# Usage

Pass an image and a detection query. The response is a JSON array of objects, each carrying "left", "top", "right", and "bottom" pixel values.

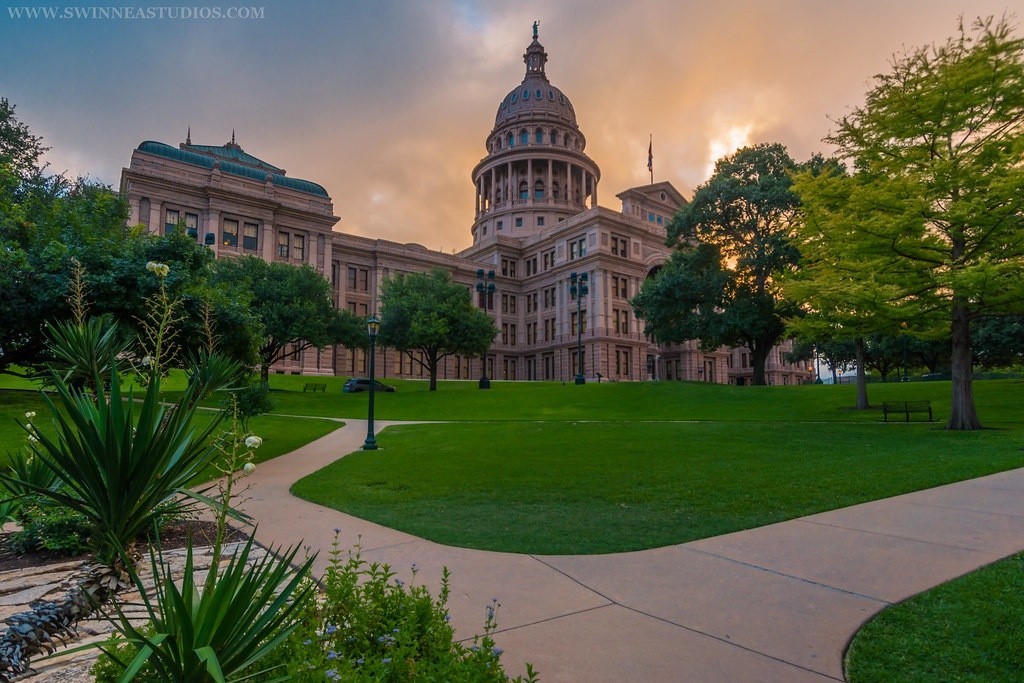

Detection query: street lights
[
  {"left": 363, "top": 316, "right": 381, "bottom": 450},
  {"left": 476, "top": 269, "right": 496, "bottom": 389},
  {"left": 570, "top": 272, "right": 588, "bottom": 384}
]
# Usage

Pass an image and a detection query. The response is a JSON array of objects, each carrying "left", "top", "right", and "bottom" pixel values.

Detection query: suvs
[{"left": 343, "top": 378, "right": 396, "bottom": 392}]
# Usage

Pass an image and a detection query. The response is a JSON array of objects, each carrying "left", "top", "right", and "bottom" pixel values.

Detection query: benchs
[
  {"left": 882, "top": 401, "right": 934, "bottom": 422},
  {"left": 303, "top": 383, "right": 326, "bottom": 393}
]
